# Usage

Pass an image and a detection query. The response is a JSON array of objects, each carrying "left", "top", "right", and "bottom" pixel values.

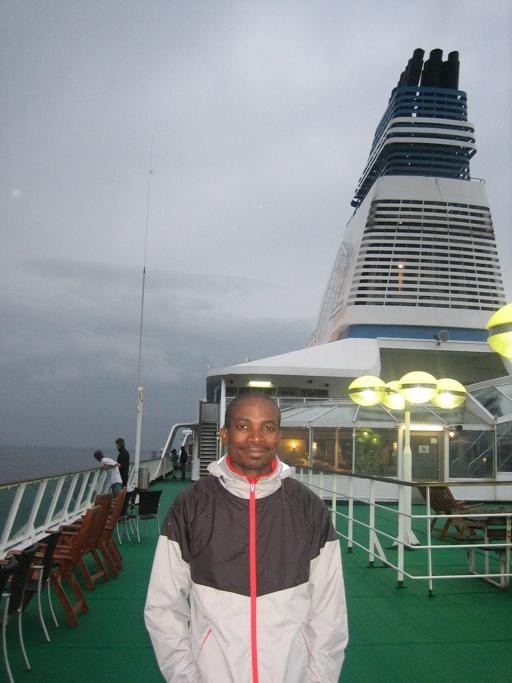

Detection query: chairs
[
  {"left": 417, "top": 480, "right": 512, "bottom": 542},
  {"left": 0, "top": 485, "right": 163, "bottom": 683}
]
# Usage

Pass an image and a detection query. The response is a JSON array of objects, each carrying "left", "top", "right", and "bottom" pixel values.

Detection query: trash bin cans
[{"left": 138, "top": 468, "right": 149, "bottom": 489}]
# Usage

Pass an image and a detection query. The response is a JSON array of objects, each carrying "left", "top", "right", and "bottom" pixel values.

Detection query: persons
[
  {"left": 94, "top": 450, "right": 124, "bottom": 497},
  {"left": 115, "top": 437, "right": 130, "bottom": 504},
  {"left": 142, "top": 390, "right": 349, "bottom": 683},
  {"left": 171, "top": 448, "right": 179, "bottom": 479},
  {"left": 179, "top": 445, "right": 188, "bottom": 479}
]
